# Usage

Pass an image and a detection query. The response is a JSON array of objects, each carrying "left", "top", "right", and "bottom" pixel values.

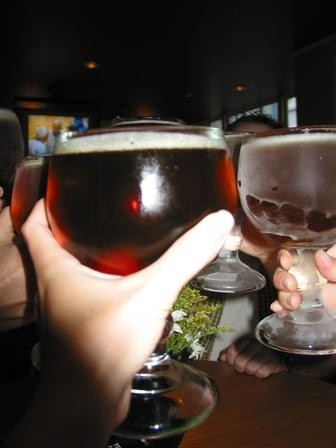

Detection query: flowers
[{"left": 158, "top": 284, "right": 239, "bottom": 361}]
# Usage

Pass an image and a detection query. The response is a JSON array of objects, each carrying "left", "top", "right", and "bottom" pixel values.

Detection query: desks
[{"left": 0, "top": 359, "right": 336, "bottom": 448}]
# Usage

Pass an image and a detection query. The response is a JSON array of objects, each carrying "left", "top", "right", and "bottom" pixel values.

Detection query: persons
[
  {"left": 46, "top": 120, "right": 66, "bottom": 155},
  {"left": 29, "top": 126, "right": 49, "bottom": 155},
  {"left": 21, "top": 197, "right": 235, "bottom": 448},
  {"left": 196, "top": 110, "right": 288, "bottom": 379}
]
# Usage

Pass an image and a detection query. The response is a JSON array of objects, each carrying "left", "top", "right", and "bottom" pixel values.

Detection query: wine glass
[
  {"left": 236, "top": 125, "right": 336, "bottom": 355},
  {"left": 41, "top": 125, "right": 237, "bottom": 441},
  {"left": 189, "top": 131, "right": 267, "bottom": 294},
  {"left": 9, "top": 154, "right": 49, "bottom": 371}
]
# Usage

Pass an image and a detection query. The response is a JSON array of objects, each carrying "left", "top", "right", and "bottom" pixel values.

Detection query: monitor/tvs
[{"left": 17, "top": 107, "right": 98, "bottom": 157}]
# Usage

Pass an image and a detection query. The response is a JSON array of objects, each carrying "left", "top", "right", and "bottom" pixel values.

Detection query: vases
[{"left": 106, "top": 376, "right": 186, "bottom": 448}]
